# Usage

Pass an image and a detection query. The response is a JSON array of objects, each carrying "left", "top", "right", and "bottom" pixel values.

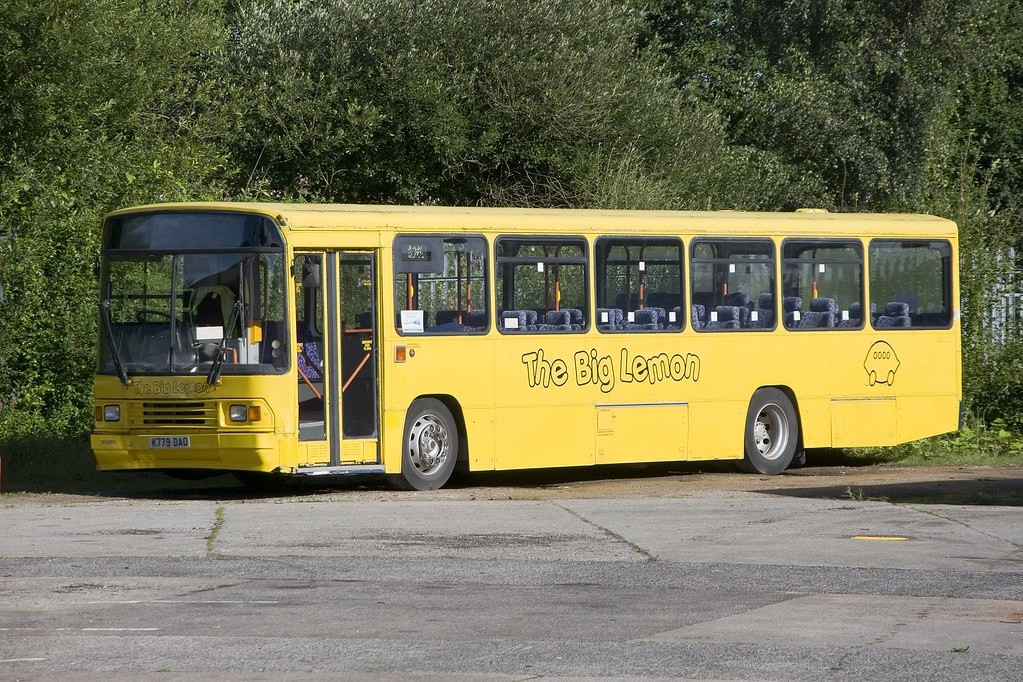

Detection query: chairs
[
  {"left": 260, "top": 319, "right": 313, "bottom": 348},
  {"left": 351, "top": 291, "right": 914, "bottom": 330},
  {"left": 189, "top": 284, "right": 237, "bottom": 339}
]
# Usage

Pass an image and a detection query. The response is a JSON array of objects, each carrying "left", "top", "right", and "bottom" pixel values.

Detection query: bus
[{"left": 90, "top": 200, "right": 964, "bottom": 490}]
